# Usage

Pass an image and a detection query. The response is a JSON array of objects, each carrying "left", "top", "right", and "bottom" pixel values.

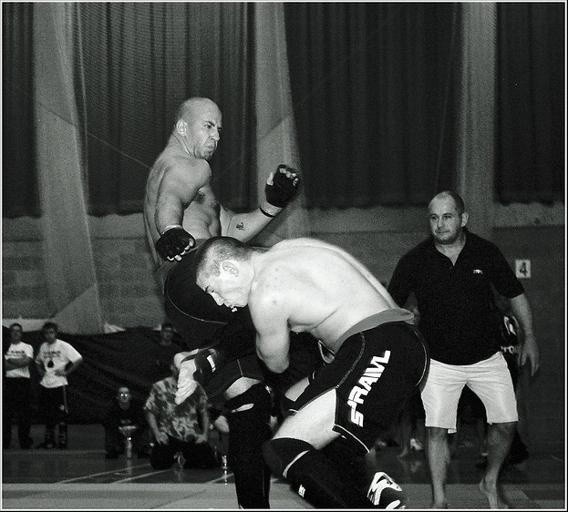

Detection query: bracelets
[{"left": 258, "top": 206, "right": 276, "bottom": 218}]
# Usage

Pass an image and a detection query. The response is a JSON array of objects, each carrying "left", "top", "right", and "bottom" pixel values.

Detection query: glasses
[{"left": 117, "top": 391, "right": 129, "bottom": 398}]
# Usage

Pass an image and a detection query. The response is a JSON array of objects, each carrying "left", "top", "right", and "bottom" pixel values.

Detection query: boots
[
  {"left": 58, "top": 431, "right": 66, "bottom": 449},
  {"left": 44, "top": 428, "right": 55, "bottom": 449}
]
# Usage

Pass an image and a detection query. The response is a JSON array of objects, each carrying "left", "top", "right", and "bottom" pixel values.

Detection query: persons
[
  {"left": 3, "top": 324, "right": 34, "bottom": 450},
  {"left": 387, "top": 191, "right": 541, "bottom": 509},
  {"left": 195, "top": 238, "right": 429, "bottom": 509},
  {"left": 365, "top": 312, "right": 532, "bottom": 463},
  {"left": 107, "top": 323, "right": 231, "bottom": 472},
  {"left": 34, "top": 322, "right": 83, "bottom": 449},
  {"left": 143, "top": 96, "right": 300, "bottom": 509}
]
